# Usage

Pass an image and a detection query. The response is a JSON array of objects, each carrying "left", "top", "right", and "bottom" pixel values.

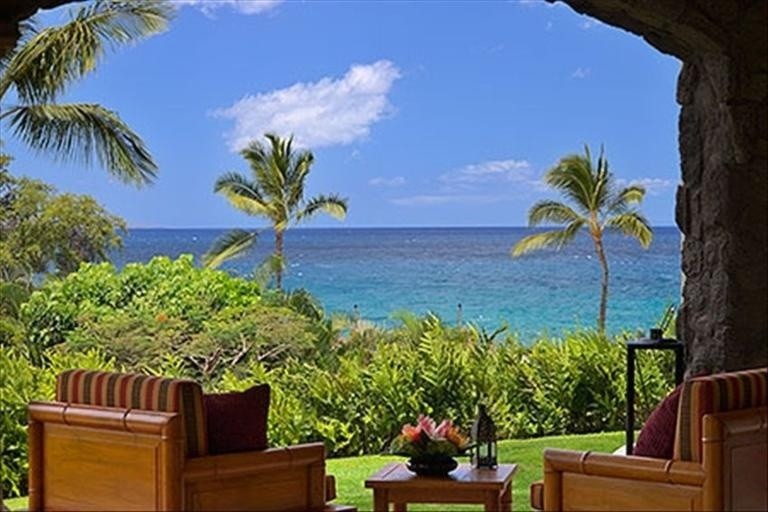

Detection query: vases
[{"left": 405, "top": 455, "right": 459, "bottom": 476}]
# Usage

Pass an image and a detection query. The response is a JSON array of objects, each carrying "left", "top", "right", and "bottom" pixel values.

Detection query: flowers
[{"left": 386, "top": 413, "right": 480, "bottom": 460}]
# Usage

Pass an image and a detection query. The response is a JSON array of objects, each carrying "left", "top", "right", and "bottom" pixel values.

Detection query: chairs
[
  {"left": 529, "top": 367, "right": 768, "bottom": 512},
  {"left": 26, "top": 366, "right": 355, "bottom": 512}
]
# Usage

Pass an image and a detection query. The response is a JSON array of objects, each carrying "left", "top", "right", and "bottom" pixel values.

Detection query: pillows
[
  {"left": 203, "top": 381, "right": 271, "bottom": 457},
  {"left": 631, "top": 383, "right": 682, "bottom": 458}
]
